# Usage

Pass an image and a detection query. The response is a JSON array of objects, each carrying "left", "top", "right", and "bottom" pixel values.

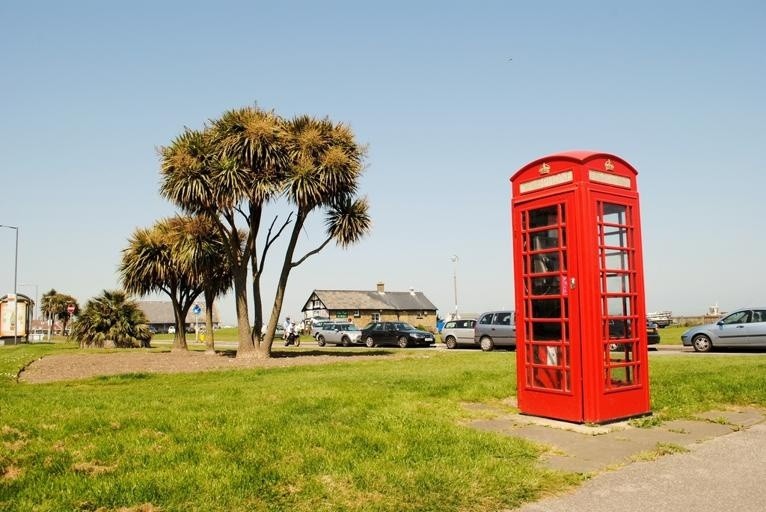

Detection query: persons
[
  {"left": 752, "top": 313, "right": 760, "bottom": 322},
  {"left": 285, "top": 321, "right": 297, "bottom": 341},
  {"left": 283, "top": 317, "right": 291, "bottom": 338}
]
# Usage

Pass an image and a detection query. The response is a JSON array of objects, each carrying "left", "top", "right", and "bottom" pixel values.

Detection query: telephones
[{"left": 533, "top": 235, "right": 561, "bottom": 287}]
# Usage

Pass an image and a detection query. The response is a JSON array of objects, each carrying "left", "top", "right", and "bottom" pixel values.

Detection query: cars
[
  {"left": 311, "top": 319, "right": 435, "bottom": 347},
  {"left": 603, "top": 315, "right": 660, "bottom": 351},
  {"left": 148, "top": 325, "right": 207, "bottom": 334},
  {"left": 260, "top": 323, "right": 287, "bottom": 340},
  {"left": 682, "top": 308, "right": 766, "bottom": 354}
]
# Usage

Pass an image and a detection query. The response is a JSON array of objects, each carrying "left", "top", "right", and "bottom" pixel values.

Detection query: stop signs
[{"left": 66, "top": 303, "right": 75, "bottom": 313}]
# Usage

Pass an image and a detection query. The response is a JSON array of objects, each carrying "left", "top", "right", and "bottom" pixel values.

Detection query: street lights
[
  {"left": 0, "top": 225, "right": 18, "bottom": 345},
  {"left": 450, "top": 254, "right": 459, "bottom": 319}
]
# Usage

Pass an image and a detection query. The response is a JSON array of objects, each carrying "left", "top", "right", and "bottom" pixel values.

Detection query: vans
[
  {"left": 441, "top": 318, "right": 480, "bottom": 349},
  {"left": 474, "top": 311, "right": 516, "bottom": 352}
]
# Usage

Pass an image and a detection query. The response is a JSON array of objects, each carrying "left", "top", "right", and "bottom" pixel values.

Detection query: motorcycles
[{"left": 287, "top": 329, "right": 300, "bottom": 346}]
